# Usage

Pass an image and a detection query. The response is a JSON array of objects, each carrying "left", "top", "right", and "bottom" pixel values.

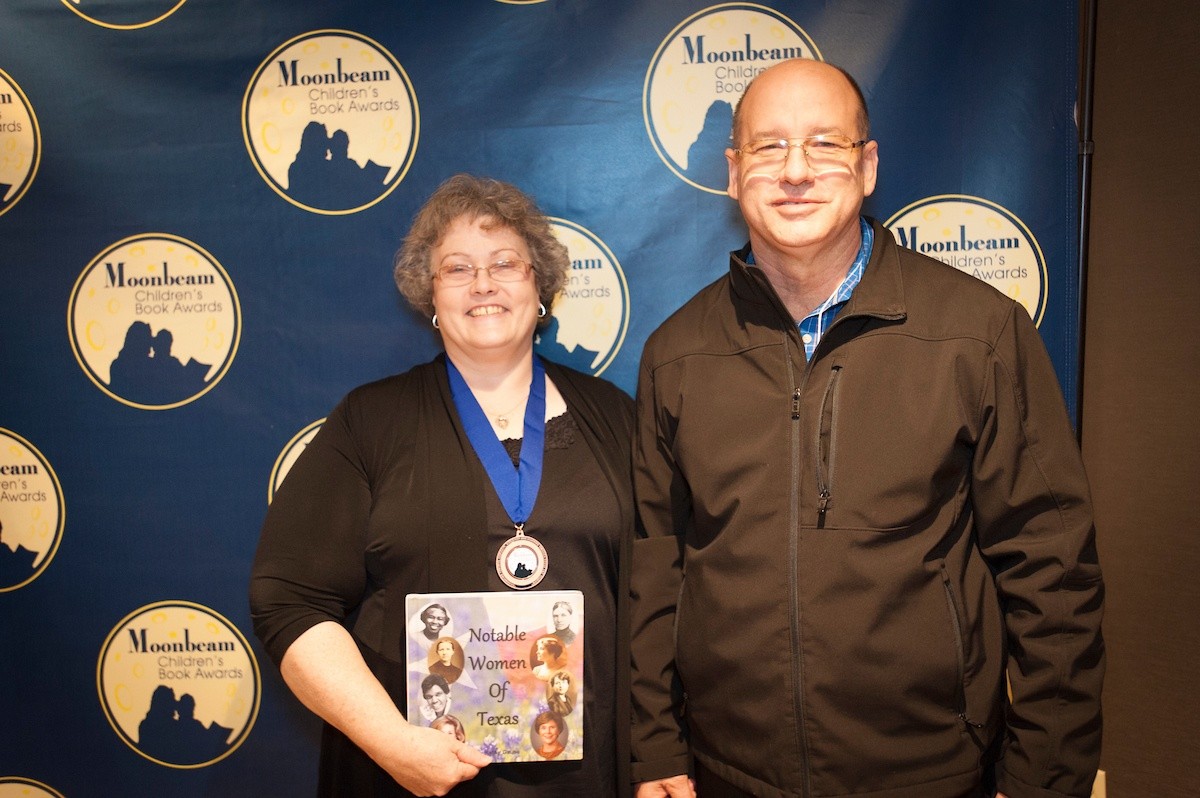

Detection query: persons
[
  {"left": 547, "top": 672, "right": 574, "bottom": 717},
  {"left": 429, "top": 639, "right": 463, "bottom": 684},
  {"left": 432, "top": 714, "right": 465, "bottom": 742},
  {"left": 632, "top": 58, "right": 1106, "bottom": 798},
  {"left": 551, "top": 601, "right": 577, "bottom": 645},
  {"left": 533, "top": 638, "right": 563, "bottom": 681},
  {"left": 251, "top": 174, "right": 634, "bottom": 798},
  {"left": 420, "top": 604, "right": 450, "bottom": 640},
  {"left": 420, "top": 672, "right": 452, "bottom": 722},
  {"left": 535, "top": 711, "right": 565, "bottom": 759}
]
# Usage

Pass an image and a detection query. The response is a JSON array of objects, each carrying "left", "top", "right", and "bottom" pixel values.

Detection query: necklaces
[{"left": 483, "top": 396, "right": 529, "bottom": 429}]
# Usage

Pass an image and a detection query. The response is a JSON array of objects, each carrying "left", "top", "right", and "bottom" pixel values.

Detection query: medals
[{"left": 495, "top": 529, "right": 548, "bottom": 590}]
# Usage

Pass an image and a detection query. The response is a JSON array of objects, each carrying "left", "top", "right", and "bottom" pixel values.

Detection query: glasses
[
  {"left": 733, "top": 136, "right": 867, "bottom": 175},
  {"left": 430, "top": 259, "right": 538, "bottom": 289}
]
